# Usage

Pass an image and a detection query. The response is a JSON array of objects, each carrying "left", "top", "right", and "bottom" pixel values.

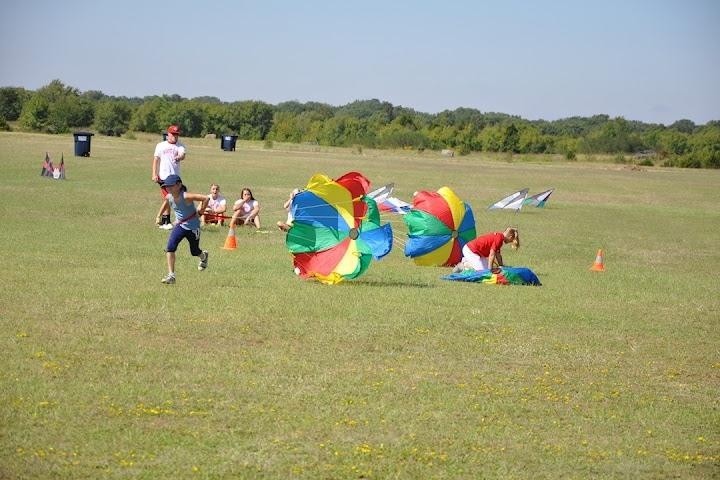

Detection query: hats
[
  {"left": 167, "top": 126, "right": 181, "bottom": 134},
  {"left": 160, "top": 175, "right": 179, "bottom": 188}
]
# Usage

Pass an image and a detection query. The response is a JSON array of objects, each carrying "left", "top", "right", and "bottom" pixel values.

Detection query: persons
[
  {"left": 451, "top": 227, "right": 520, "bottom": 277},
  {"left": 196, "top": 183, "right": 227, "bottom": 228},
  {"left": 276, "top": 187, "right": 301, "bottom": 235},
  {"left": 151, "top": 125, "right": 187, "bottom": 225},
  {"left": 228, "top": 188, "right": 265, "bottom": 230},
  {"left": 155, "top": 174, "right": 212, "bottom": 286}
]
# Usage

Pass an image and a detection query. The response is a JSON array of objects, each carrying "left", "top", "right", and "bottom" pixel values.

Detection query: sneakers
[
  {"left": 161, "top": 275, "right": 175, "bottom": 284},
  {"left": 198, "top": 250, "right": 208, "bottom": 271},
  {"left": 159, "top": 223, "right": 173, "bottom": 230}
]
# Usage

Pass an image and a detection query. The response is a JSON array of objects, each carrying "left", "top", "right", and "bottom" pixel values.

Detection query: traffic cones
[
  {"left": 219, "top": 223, "right": 239, "bottom": 251},
  {"left": 588, "top": 248, "right": 608, "bottom": 272}
]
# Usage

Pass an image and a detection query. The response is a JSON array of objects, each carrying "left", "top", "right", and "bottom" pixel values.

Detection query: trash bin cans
[
  {"left": 74, "top": 132, "right": 94, "bottom": 157},
  {"left": 221, "top": 134, "right": 238, "bottom": 151},
  {"left": 163, "top": 133, "right": 168, "bottom": 141}
]
